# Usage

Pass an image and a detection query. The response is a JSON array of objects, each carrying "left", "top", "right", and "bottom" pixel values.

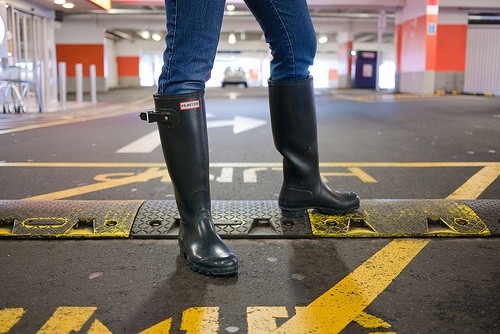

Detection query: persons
[{"left": 138, "top": 0, "right": 361, "bottom": 276}]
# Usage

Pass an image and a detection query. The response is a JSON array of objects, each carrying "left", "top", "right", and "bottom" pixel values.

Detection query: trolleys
[{"left": 2, "top": 59, "right": 44, "bottom": 113}]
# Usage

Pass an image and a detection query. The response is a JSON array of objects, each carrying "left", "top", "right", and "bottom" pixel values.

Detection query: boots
[
  {"left": 266, "top": 76, "right": 361, "bottom": 216},
  {"left": 140, "top": 91, "right": 239, "bottom": 278}
]
